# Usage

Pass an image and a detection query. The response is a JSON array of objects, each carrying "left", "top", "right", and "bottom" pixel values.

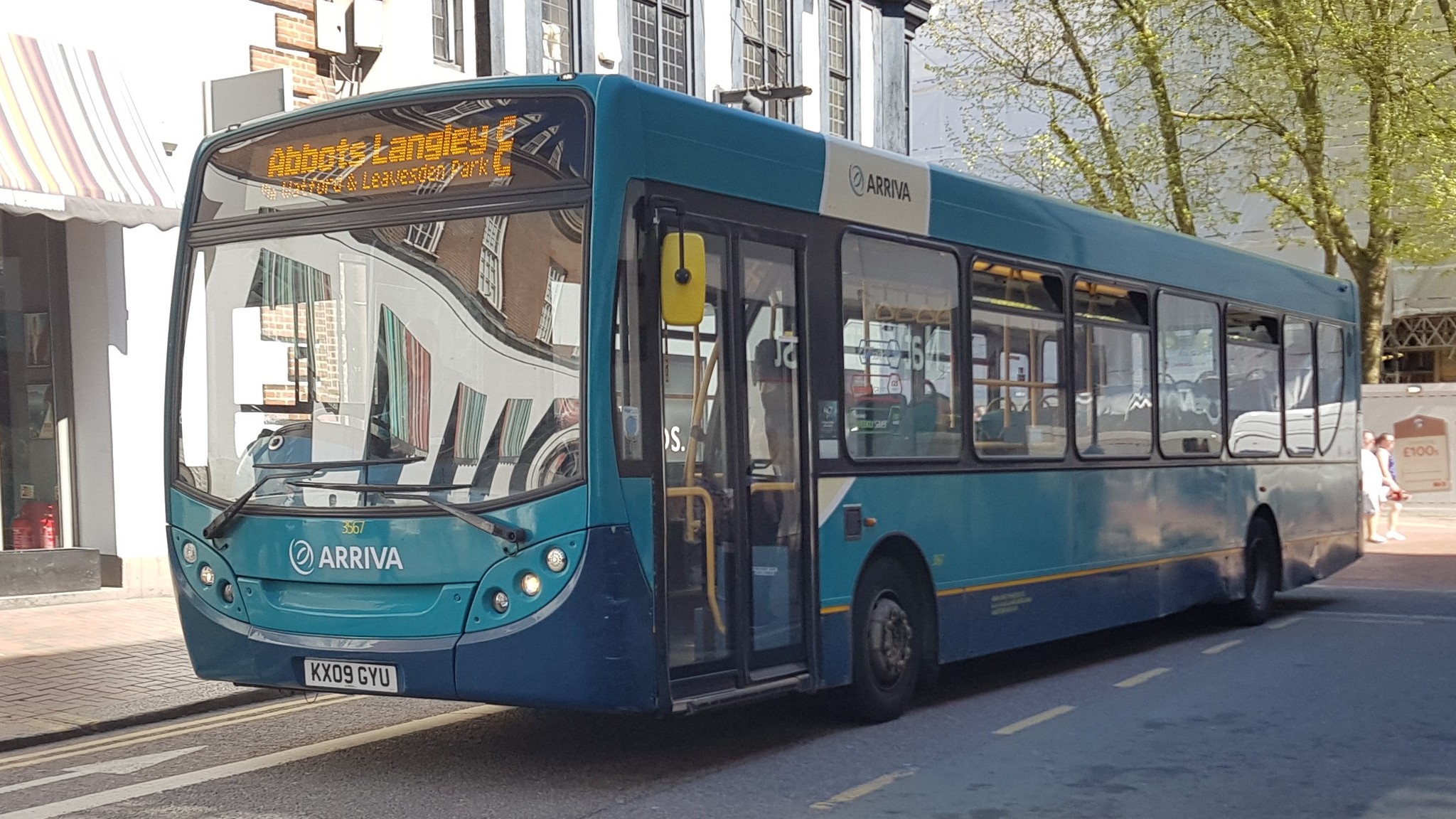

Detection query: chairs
[{"left": 851, "top": 366, "right": 1274, "bottom": 459}]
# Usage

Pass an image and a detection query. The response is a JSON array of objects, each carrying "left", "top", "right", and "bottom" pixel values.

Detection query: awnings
[{"left": 0, "top": 29, "right": 184, "bottom": 232}]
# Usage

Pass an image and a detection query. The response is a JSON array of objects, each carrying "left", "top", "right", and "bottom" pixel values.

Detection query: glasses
[
  {"left": 1370, "top": 438, "right": 1376, "bottom": 442},
  {"left": 1386, "top": 440, "right": 1395, "bottom": 443}
]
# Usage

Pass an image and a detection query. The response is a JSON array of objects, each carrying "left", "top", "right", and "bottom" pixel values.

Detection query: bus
[{"left": 158, "top": 71, "right": 1365, "bottom": 724}]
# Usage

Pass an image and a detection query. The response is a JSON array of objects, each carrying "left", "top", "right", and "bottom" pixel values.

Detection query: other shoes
[
  {"left": 1366, "top": 533, "right": 1386, "bottom": 543},
  {"left": 1387, "top": 530, "right": 1407, "bottom": 542}
]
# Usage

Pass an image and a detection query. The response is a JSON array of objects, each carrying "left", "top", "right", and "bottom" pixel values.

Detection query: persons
[
  {"left": 1372, "top": 432, "right": 1407, "bottom": 541},
  {"left": 1360, "top": 431, "right": 1391, "bottom": 542}
]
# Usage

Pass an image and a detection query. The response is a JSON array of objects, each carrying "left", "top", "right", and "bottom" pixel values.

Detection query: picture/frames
[{"left": 22, "top": 312, "right": 55, "bottom": 439}]
